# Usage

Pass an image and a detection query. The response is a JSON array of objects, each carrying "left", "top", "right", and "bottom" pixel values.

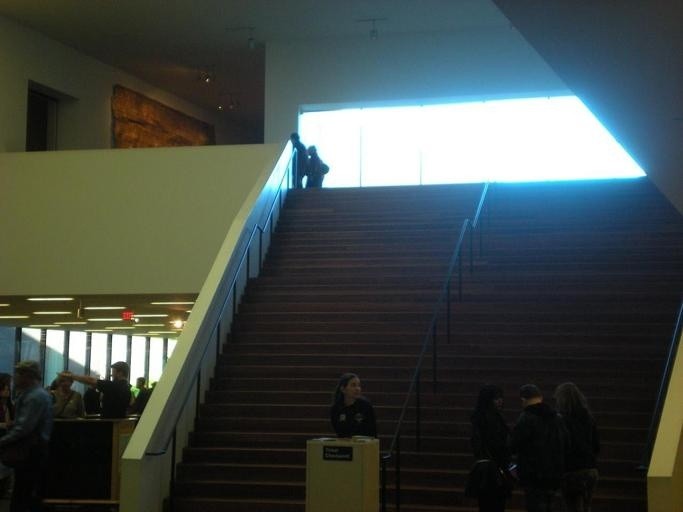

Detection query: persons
[
  {"left": 304, "top": 145, "right": 323, "bottom": 188},
  {"left": 464, "top": 383, "right": 514, "bottom": 511},
  {"left": 504, "top": 382, "right": 563, "bottom": 512},
  {"left": 45, "top": 360, "right": 156, "bottom": 417},
  {"left": 328, "top": 372, "right": 378, "bottom": 438},
  {"left": 0, "top": 373, "right": 16, "bottom": 512},
  {"left": 0, "top": 357, "right": 53, "bottom": 512},
  {"left": 290, "top": 133, "right": 307, "bottom": 188},
  {"left": 552, "top": 382, "right": 603, "bottom": 512}
]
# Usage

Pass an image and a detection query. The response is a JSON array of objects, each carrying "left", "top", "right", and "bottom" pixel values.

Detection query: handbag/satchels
[{"left": 463, "top": 460, "right": 499, "bottom": 501}]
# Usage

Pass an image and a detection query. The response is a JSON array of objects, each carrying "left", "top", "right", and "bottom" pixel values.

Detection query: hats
[
  {"left": 111, "top": 363, "right": 129, "bottom": 373},
  {"left": 12, "top": 362, "right": 39, "bottom": 375}
]
